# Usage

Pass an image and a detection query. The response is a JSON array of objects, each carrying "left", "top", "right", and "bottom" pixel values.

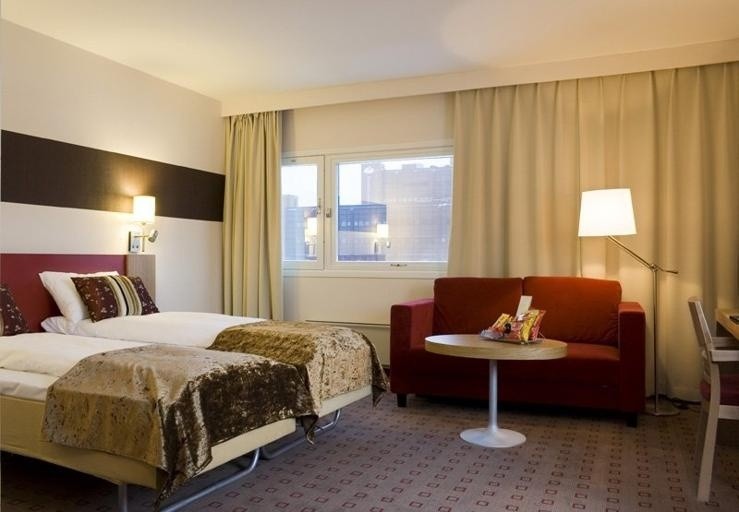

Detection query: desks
[{"left": 715, "top": 306, "right": 739, "bottom": 341}]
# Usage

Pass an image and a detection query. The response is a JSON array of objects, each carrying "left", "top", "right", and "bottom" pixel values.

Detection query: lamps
[
  {"left": 129, "top": 230, "right": 159, "bottom": 253},
  {"left": 577, "top": 187, "right": 680, "bottom": 415},
  {"left": 133, "top": 195, "right": 156, "bottom": 253}
]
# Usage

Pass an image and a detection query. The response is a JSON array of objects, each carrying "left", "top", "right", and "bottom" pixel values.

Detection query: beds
[
  {"left": 0, "top": 332, "right": 322, "bottom": 512},
  {"left": 41, "top": 311, "right": 390, "bottom": 459}
]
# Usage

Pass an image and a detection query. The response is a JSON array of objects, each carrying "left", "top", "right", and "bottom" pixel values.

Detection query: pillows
[
  {"left": 39, "top": 270, "right": 120, "bottom": 324},
  {"left": 0, "top": 282, "right": 32, "bottom": 337},
  {"left": 71, "top": 275, "right": 159, "bottom": 323}
]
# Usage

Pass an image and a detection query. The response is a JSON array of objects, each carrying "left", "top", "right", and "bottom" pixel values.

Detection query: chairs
[{"left": 688, "top": 300, "right": 739, "bottom": 502}]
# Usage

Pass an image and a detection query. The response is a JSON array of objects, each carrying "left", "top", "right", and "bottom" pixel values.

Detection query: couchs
[{"left": 390, "top": 276, "right": 646, "bottom": 428}]
